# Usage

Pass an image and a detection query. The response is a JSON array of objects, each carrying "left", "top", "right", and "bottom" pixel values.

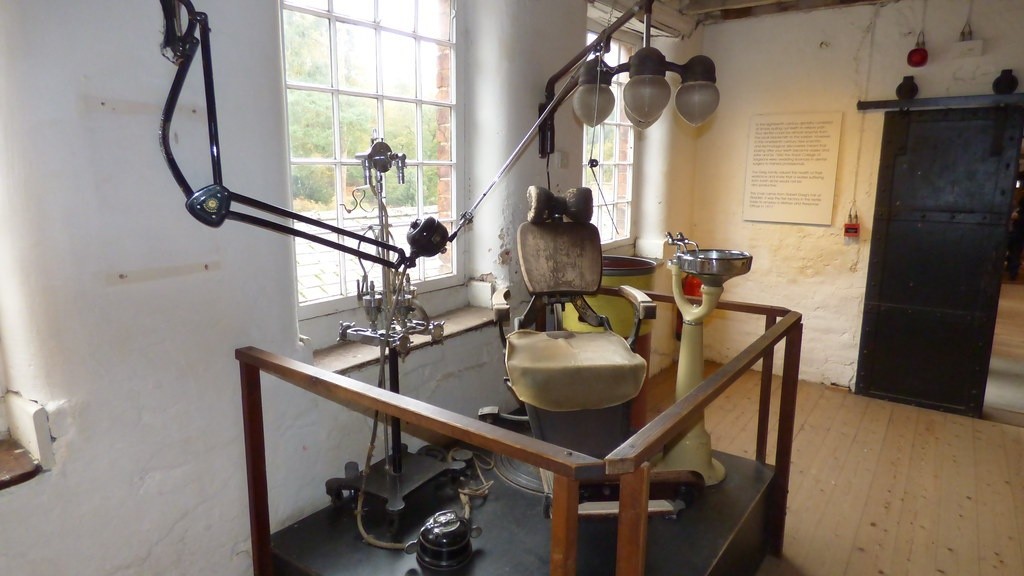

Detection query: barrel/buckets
[{"left": 559, "top": 250, "right": 658, "bottom": 431}]
